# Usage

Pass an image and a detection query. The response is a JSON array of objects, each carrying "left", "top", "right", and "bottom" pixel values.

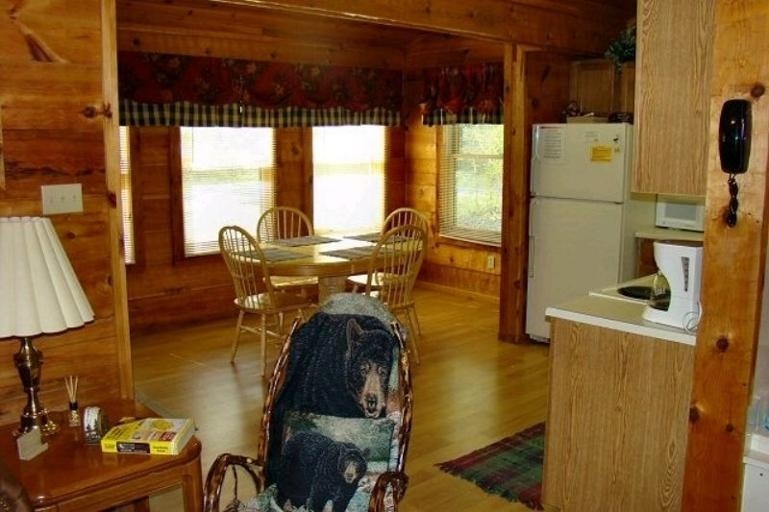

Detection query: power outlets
[{"left": 40, "top": 183, "right": 85, "bottom": 216}]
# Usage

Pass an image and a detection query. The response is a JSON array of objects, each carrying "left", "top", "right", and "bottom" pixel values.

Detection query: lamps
[{"left": 0, "top": 216, "right": 95, "bottom": 437}]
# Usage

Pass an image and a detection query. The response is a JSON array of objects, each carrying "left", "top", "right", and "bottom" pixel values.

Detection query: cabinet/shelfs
[{"left": 540, "top": 1, "right": 769, "bottom": 512}]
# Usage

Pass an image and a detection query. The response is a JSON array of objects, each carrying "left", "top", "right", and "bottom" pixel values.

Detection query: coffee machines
[{"left": 641, "top": 239, "right": 703, "bottom": 331}]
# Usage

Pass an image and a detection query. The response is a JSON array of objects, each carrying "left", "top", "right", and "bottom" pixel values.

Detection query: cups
[{"left": 83, "top": 407, "right": 102, "bottom": 446}]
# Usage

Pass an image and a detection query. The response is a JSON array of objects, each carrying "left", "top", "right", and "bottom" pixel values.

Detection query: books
[{"left": 99, "top": 414, "right": 196, "bottom": 457}]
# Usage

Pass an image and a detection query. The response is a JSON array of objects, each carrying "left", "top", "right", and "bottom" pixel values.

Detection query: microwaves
[{"left": 654, "top": 192, "right": 706, "bottom": 231}]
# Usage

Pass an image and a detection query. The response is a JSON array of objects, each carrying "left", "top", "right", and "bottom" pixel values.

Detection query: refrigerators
[{"left": 523, "top": 122, "right": 657, "bottom": 343}]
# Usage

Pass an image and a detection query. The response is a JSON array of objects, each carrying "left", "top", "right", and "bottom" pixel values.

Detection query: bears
[
  {"left": 286, "top": 311, "right": 399, "bottom": 419},
  {"left": 274, "top": 431, "right": 371, "bottom": 512}
]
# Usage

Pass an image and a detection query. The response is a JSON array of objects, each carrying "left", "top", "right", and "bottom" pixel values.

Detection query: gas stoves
[{"left": 589, "top": 271, "right": 658, "bottom": 304}]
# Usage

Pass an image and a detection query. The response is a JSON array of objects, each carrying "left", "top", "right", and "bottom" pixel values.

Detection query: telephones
[{"left": 719, "top": 100, "right": 752, "bottom": 173}]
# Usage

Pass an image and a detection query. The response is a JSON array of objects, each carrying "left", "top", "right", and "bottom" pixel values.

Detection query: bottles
[{"left": 66, "top": 401, "right": 82, "bottom": 428}]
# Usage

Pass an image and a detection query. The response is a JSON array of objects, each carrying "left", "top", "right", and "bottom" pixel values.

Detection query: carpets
[{"left": 432, "top": 421, "right": 546, "bottom": 511}]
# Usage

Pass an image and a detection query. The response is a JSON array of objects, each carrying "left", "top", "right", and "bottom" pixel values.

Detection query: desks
[{"left": 1, "top": 398, "right": 204, "bottom": 511}]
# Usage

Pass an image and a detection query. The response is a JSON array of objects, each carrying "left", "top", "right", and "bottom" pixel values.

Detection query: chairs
[
  {"left": 219, "top": 206, "right": 429, "bottom": 377},
  {"left": 207, "top": 293, "right": 414, "bottom": 512}
]
